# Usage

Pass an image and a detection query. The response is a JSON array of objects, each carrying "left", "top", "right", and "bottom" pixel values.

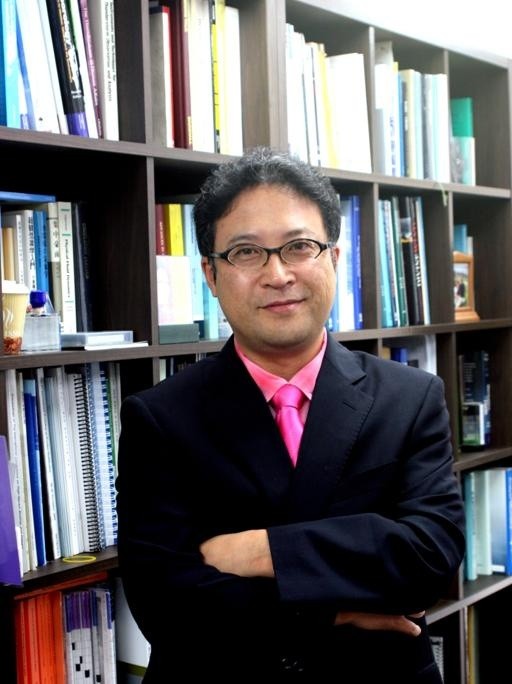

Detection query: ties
[{"left": 272, "top": 384, "right": 305, "bottom": 468}]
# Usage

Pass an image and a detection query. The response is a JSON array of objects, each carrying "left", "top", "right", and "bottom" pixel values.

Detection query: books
[
  {"left": 347, "top": 334, "right": 492, "bottom": 452},
  {"left": 451, "top": 464, "right": 511, "bottom": 585},
  {"left": 429, "top": 606, "right": 478, "bottom": 684},
  {"left": 151, "top": 195, "right": 479, "bottom": 343},
  {"left": 1, "top": 2, "right": 477, "bottom": 188},
  {"left": 0, "top": 192, "right": 153, "bottom": 684}
]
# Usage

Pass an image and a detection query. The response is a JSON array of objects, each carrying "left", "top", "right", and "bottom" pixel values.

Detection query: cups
[{"left": 0, "top": 280, "right": 30, "bottom": 354}]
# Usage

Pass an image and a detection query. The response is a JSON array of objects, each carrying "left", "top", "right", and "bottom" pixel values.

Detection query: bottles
[{"left": 21, "top": 291, "right": 63, "bottom": 355}]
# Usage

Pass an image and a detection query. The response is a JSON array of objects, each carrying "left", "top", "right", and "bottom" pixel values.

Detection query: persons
[{"left": 119, "top": 143, "right": 466, "bottom": 683}]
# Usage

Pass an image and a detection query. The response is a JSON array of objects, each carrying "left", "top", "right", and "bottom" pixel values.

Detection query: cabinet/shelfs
[{"left": 0, "top": 0, "right": 510, "bottom": 683}]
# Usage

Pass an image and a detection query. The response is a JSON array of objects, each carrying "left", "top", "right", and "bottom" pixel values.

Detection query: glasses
[{"left": 210, "top": 238, "right": 334, "bottom": 271}]
